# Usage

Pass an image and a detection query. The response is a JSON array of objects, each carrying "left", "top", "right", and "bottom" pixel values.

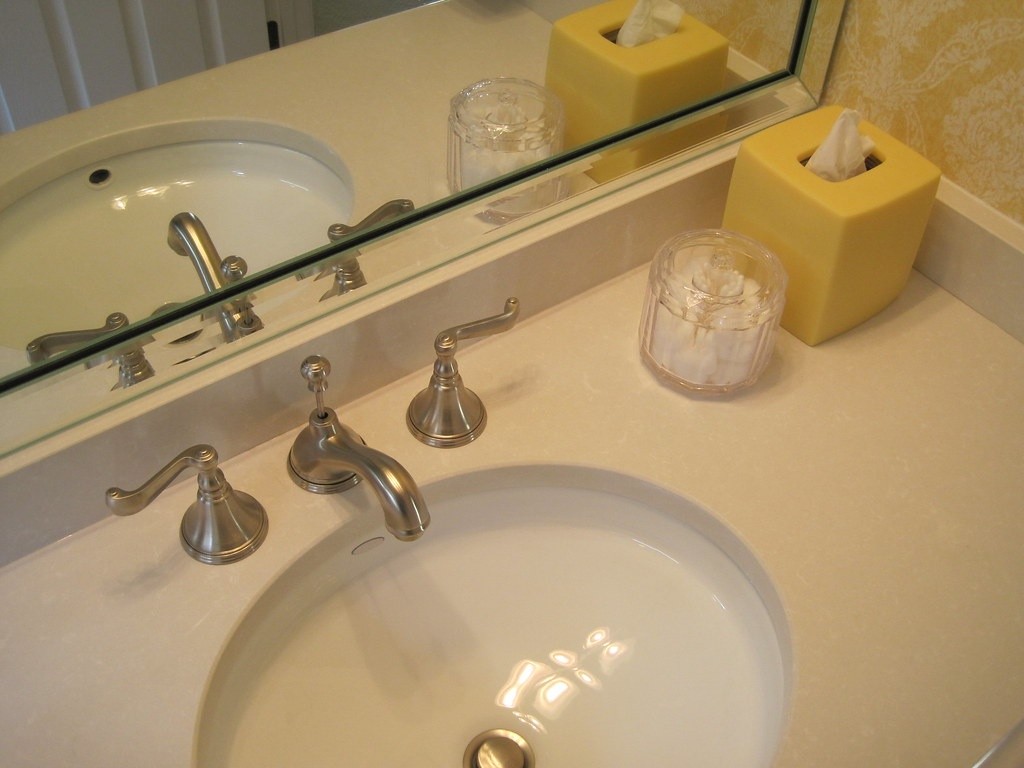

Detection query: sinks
[
  {"left": 187, "top": 459, "right": 799, "bottom": 768},
  {"left": 0, "top": 115, "right": 360, "bottom": 376}
]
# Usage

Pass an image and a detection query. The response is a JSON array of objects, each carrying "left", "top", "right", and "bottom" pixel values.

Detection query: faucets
[
  {"left": 164, "top": 209, "right": 244, "bottom": 300},
  {"left": 286, "top": 351, "right": 433, "bottom": 546}
]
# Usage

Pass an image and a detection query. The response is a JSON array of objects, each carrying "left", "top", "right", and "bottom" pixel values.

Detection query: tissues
[
  {"left": 541, "top": 0, "right": 731, "bottom": 176},
  {"left": 719, "top": 99, "right": 943, "bottom": 345}
]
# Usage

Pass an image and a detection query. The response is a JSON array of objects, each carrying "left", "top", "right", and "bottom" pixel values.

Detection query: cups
[
  {"left": 637, "top": 225, "right": 790, "bottom": 400},
  {"left": 448, "top": 78, "right": 568, "bottom": 197}
]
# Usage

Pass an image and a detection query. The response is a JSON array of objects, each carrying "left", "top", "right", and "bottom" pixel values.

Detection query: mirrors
[{"left": 0, "top": 2, "right": 814, "bottom": 386}]
